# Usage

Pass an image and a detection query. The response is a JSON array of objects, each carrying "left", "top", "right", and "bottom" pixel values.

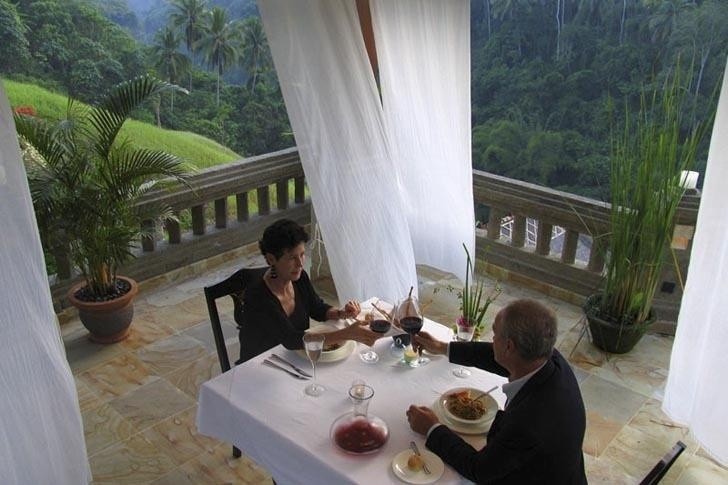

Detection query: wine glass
[
  {"left": 359, "top": 297, "right": 395, "bottom": 364},
  {"left": 303, "top": 333, "right": 326, "bottom": 398},
  {"left": 397, "top": 295, "right": 432, "bottom": 368},
  {"left": 452, "top": 317, "right": 478, "bottom": 378}
]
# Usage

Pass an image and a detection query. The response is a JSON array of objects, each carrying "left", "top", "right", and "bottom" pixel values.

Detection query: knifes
[
  {"left": 410, "top": 441, "right": 431, "bottom": 474},
  {"left": 264, "top": 359, "right": 310, "bottom": 380},
  {"left": 272, "top": 353, "right": 312, "bottom": 377}
]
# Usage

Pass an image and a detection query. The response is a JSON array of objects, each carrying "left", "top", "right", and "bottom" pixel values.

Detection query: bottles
[{"left": 328, "top": 385, "right": 390, "bottom": 457}]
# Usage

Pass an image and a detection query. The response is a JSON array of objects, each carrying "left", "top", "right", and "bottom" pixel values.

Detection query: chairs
[
  {"left": 204, "top": 266, "right": 269, "bottom": 458},
  {"left": 583, "top": 439, "right": 688, "bottom": 485}
]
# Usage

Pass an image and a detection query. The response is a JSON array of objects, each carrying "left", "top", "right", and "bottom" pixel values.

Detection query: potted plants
[
  {"left": 10, "top": 71, "right": 203, "bottom": 344},
  {"left": 559, "top": 51, "right": 721, "bottom": 376},
  {"left": 451, "top": 242, "right": 504, "bottom": 341}
]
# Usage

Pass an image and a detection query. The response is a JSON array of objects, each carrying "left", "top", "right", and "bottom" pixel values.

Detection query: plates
[
  {"left": 392, "top": 449, "right": 445, "bottom": 485},
  {"left": 296, "top": 324, "right": 357, "bottom": 362},
  {"left": 434, "top": 398, "right": 502, "bottom": 435}
]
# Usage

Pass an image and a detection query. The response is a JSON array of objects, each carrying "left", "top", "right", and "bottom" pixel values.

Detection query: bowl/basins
[
  {"left": 304, "top": 325, "right": 344, "bottom": 353},
  {"left": 439, "top": 387, "right": 498, "bottom": 425}
]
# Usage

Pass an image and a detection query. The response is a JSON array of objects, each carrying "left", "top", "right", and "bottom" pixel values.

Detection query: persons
[
  {"left": 406, "top": 299, "right": 588, "bottom": 485},
  {"left": 235, "top": 218, "right": 385, "bottom": 365}
]
